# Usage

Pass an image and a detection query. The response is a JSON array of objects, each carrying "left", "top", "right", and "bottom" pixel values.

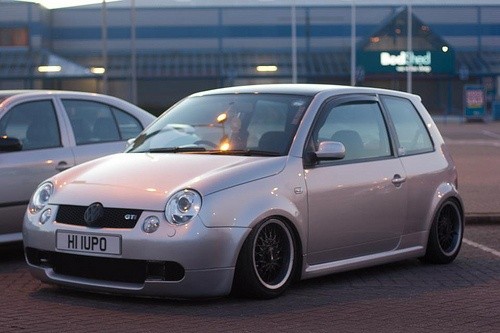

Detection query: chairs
[
  {"left": 24, "top": 114, "right": 117, "bottom": 147},
  {"left": 331, "top": 130, "right": 362, "bottom": 159},
  {"left": 258, "top": 131, "right": 285, "bottom": 152}
]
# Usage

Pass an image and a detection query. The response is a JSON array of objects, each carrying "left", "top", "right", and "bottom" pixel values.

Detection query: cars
[
  {"left": 0, "top": 89, "right": 157, "bottom": 243},
  {"left": 22, "top": 83, "right": 466, "bottom": 305}
]
